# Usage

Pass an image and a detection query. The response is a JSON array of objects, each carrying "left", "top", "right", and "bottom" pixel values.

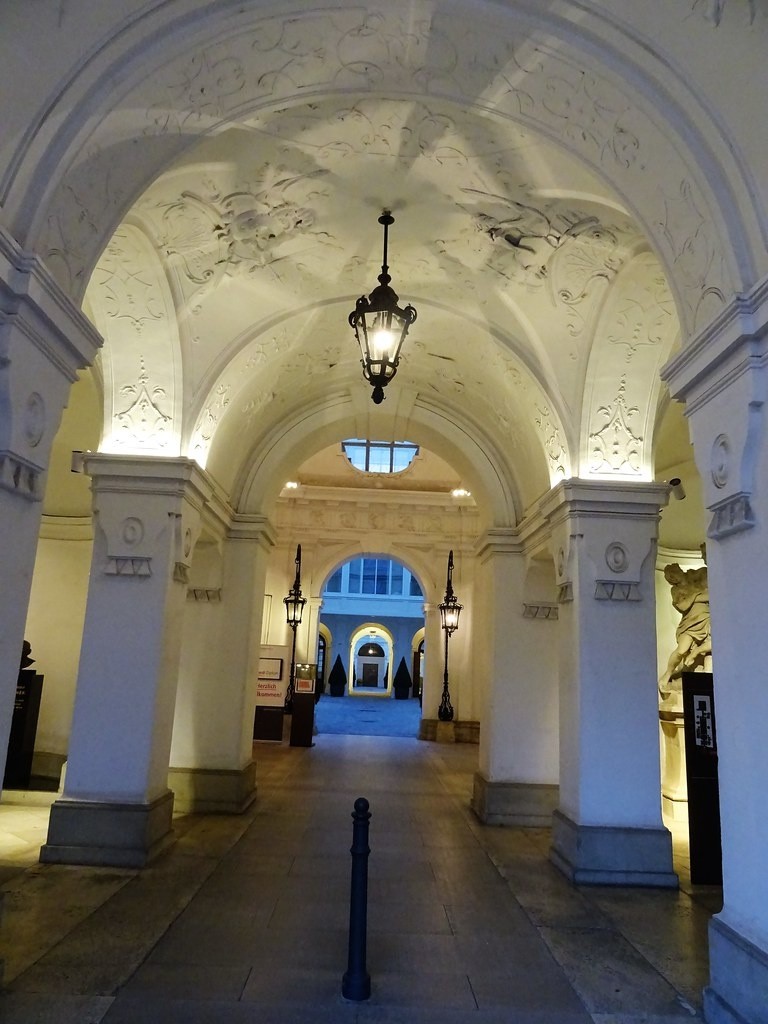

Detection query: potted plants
[
  {"left": 393, "top": 656, "right": 413, "bottom": 700},
  {"left": 327, "top": 654, "right": 347, "bottom": 697}
]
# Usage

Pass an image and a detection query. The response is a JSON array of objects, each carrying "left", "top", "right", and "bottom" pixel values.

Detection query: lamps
[
  {"left": 283, "top": 544, "right": 307, "bottom": 713},
  {"left": 348, "top": 211, "right": 418, "bottom": 404},
  {"left": 437, "top": 550, "right": 465, "bottom": 721}
]
